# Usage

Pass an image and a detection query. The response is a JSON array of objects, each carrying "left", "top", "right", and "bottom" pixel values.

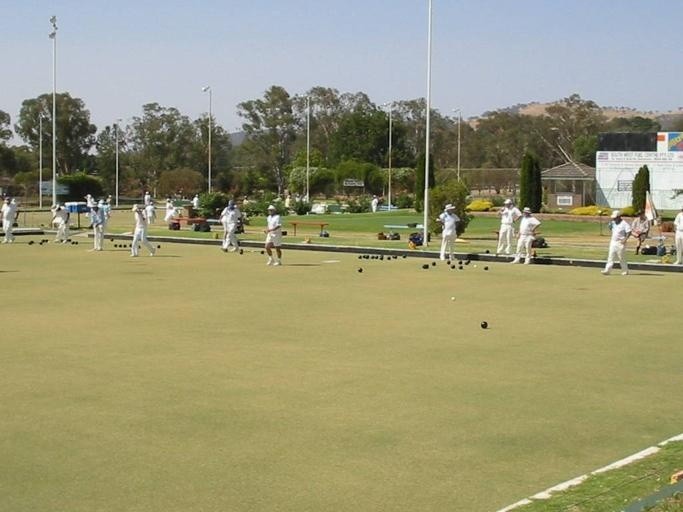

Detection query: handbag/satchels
[{"left": 641, "top": 245, "right": 665, "bottom": 255}]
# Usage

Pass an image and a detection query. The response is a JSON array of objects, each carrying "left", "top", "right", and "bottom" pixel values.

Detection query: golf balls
[
  {"left": 474, "top": 265, "right": 477, "bottom": 268},
  {"left": 484, "top": 266, "right": 488, "bottom": 270},
  {"left": 28, "top": 238, "right": 78, "bottom": 245},
  {"left": 423, "top": 258, "right": 470, "bottom": 270},
  {"left": 486, "top": 250, "right": 489, "bottom": 253},
  {"left": 261, "top": 251, "right": 264, "bottom": 254},
  {"left": 157, "top": 245, "right": 160, "bottom": 248},
  {"left": 451, "top": 297, "right": 455, "bottom": 302},
  {"left": 114, "top": 244, "right": 141, "bottom": 249},
  {"left": 111, "top": 238, "right": 114, "bottom": 241},
  {"left": 358, "top": 267, "right": 364, "bottom": 272},
  {"left": 239, "top": 249, "right": 243, "bottom": 254},
  {"left": 481, "top": 322, "right": 488, "bottom": 328},
  {"left": 358, "top": 254, "right": 406, "bottom": 260}
]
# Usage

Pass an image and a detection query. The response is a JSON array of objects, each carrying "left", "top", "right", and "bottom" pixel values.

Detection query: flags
[{"left": 644, "top": 191, "right": 657, "bottom": 221}]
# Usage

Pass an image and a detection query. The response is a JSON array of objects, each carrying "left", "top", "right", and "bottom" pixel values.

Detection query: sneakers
[
  {"left": 94, "top": 245, "right": 102, "bottom": 250},
  {"left": 1, "top": 237, "right": 15, "bottom": 243},
  {"left": 440, "top": 255, "right": 457, "bottom": 260},
  {"left": 673, "top": 259, "right": 680, "bottom": 265},
  {"left": 221, "top": 244, "right": 239, "bottom": 252},
  {"left": 600, "top": 269, "right": 610, "bottom": 275},
  {"left": 131, "top": 248, "right": 156, "bottom": 256},
  {"left": 509, "top": 256, "right": 531, "bottom": 264},
  {"left": 267, "top": 257, "right": 281, "bottom": 266},
  {"left": 621, "top": 271, "right": 628, "bottom": 275}
]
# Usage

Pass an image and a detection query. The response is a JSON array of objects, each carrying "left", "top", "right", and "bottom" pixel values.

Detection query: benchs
[
  {"left": 287, "top": 221, "right": 328, "bottom": 237},
  {"left": 167, "top": 217, "right": 210, "bottom": 232},
  {"left": 384, "top": 224, "right": 430, "bottom": 241},
  {"left": 634, "top": 236, "right": 667, "bottom": 252},
  {"left": 492, "top": 230, "right": 541, "bottom": 246}
]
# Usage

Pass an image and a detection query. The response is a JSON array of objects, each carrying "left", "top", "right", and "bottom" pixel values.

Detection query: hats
[
  {"left": 521, "top": 207, "right": 532, "bottom": 215},
  {"left": 51, "top": 203, "right": 59, "bottom": 212},
  {"left": 267, "top": 205, "right": 277, "bottom": 212},
  {"left": 443, "top": 203, "right": 456, "bottom": 212},
  {"left": 87, "top": 202, "right": 98, "bottom": 208},
  {"left": 610, "top": 210, "right": 621, "bottom": 219},
  {"left": 504, "top": 198, "right": 513, "bottom": 205}
]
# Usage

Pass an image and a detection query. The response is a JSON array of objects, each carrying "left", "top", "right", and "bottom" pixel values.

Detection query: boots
[{"left": 635, "top": 246, "right": 640, "bottom": 255}]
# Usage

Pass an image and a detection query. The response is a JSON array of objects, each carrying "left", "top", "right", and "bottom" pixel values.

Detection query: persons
[
  {"left": 272, "top": 193, "right": 308, "bottom": 214},
  {"left": 221, "top": 200, "right": 244, "bottom": 255},
  {"left": 672, "top": 209, "right": 683, "bottom": 264},
  {"left": 0, "top": 196, "right": 19, "bottom": 243},
  {"left": 47, "top": 204, "right": 69, "bottom": 244},
  {"left": 630, "top": 215, "right": 650, "bottom": 255},
  {"left": 85, "top": 194, "right": 113, "bottom": 251},
  {"left": 509, "top": 206, "right": 541, "bottom": 265},
  {"left": 263, "top": 205, "right": 282, "bottom": 265},
  {"left": 600, "top": 210, "right": 632, "bottom": 275},
  {"left": 130, "top": 190, "right": 199, "bottom": 257},
  {"left": 496, "top": 198, "right": 521, "bottom": 255},
  {"left": 439, "top": 204, "right": 460, "bottom": 261},
  {"left": 371, "top": 195, "right": 378, "bottom": 214}
]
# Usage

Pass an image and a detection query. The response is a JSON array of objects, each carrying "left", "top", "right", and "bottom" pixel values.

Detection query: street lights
[
  {"left": 380, "top": 99, "right": 397, "bottom": 211},
  {"left": 36, "top": 107, "right": 46, "bottom": 209},
  {"left": 48, "top": 11, "right": 61, "bottom": 211},
  {"left": 201, "top": 82, "right": 214, "bottom": 195},
  {"left": 448, "top": 106, "right": 462, "bottom": 183},
  {"left": 114, "top": 114, "right": 122, "bottom": 207},
  {"left": 297, "top": 90, "right": 322, "bottom": 203}
]
